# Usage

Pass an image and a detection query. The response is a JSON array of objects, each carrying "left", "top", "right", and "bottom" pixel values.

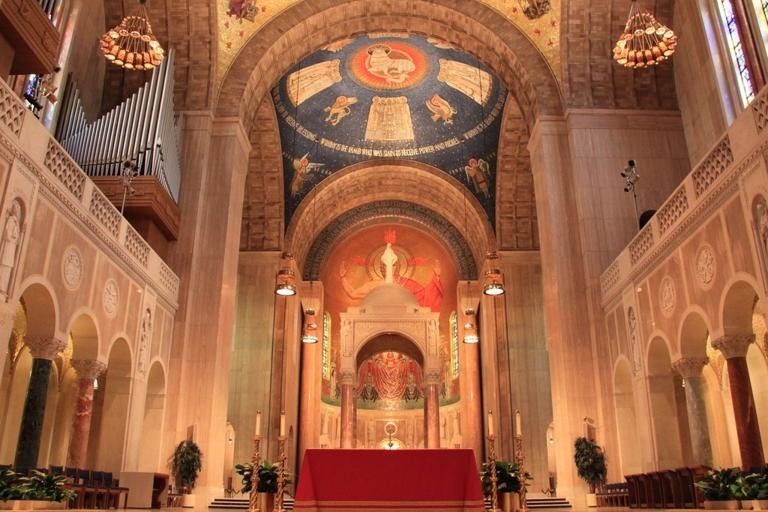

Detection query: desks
[{"left": 302, "top": 448, "right": 482, "bottom": 510}]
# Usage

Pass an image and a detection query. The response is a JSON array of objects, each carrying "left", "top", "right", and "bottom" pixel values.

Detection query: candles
[
  {"left": 254, "top": 413, "right": 261, "bottom": 437},
  {"left": 515, "top": 412, "right": 522, "bottom": 434},
  {"left": 280, "top": 414, "right": 286, "bottom": 435},
  {"left": 488, "top": 413, "right": 494, "bottom": 436}
]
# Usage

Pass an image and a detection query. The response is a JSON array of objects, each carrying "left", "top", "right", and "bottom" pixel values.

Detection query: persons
[
  {"left": 289, "top": 156, "right": 309, "bottom": 199},
  {"left": 0, "top": 199, "right": 23, "bottom": 299},
  {"left": 753, "top": 200, "right": 767, "bottom": 251},
  {"left": 377, "top": 352, "right": 405, "bottom": 365},
  {"left": 466, "top": 158, "right": 492, "bottom": 201},
  {"left": 338, "top": 255, "right": 447, "bottom": 316}
]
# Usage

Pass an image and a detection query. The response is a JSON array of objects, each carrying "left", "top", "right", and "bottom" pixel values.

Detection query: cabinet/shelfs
[{"left": 119, "top": 471, "right": 169, "bottom": 508}]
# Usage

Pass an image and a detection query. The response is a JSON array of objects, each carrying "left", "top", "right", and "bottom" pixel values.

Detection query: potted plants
[
  {"left": 481, "top": 456, "right": 535, "bottom": 511},
  {"left": 0, "top": 461, "right": 77, "bottom": 510},
  {"left": 169, "top": 438, "right": 203, "bottom": 509},
  {"left": 574, "top": 436, "right": 607, "bottom": 505},
  {"left": 233, "top": 458, "right": 295, "bottom": 511},
  {"left": 696, "top": 466, "right": 767, "bottom": 510}
]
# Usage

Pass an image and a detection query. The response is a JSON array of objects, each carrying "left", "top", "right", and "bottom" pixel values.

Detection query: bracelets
[{"left": 340, "top": 275, "right": 346, "bottom": 279}]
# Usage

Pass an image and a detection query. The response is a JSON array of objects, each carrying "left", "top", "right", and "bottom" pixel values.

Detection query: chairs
[
  {"left": 49, "top": 463, "right": 130, "bottom": 509},
  {"left": 595, "top": 483, "right": 628, "bottom": 507}
]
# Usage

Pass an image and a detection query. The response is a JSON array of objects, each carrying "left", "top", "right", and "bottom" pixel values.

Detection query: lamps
[
  {"left": 274, "top": 61, "right": 320, "bottom": 346},
  {"left": 38, "top": 73, "right": 60, "bottom": 107},
  {"left": 610, "top": 1, "right": 678, "bottom": 70},
  {"left": 460, "top": 64, "right": 507, "bottom": 344},
  {"left": 98, "top": 0, "right": 165, "bottom": 71}
]
